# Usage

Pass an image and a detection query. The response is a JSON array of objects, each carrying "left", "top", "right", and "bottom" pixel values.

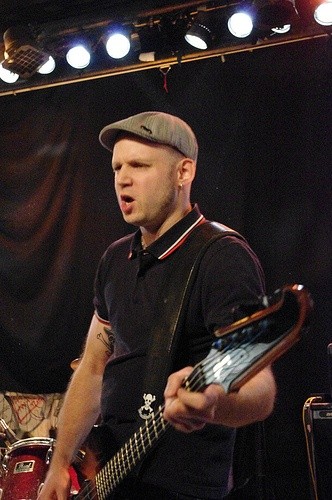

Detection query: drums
[{"left": 1, "top": 439, "right": 85, "bottom": 500}]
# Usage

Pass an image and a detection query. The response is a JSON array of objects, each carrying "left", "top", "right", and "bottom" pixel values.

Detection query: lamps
[
  {"left": 184, "top": 10, "right": 217, "bottom": 50},
  {"left": 0, "top": 26, "right": 50, "bottom": 79}
]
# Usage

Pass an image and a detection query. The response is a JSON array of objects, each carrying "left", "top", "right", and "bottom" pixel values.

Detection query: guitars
[{"left": 72, "top": 283, "right": 315, "bottom": 500}]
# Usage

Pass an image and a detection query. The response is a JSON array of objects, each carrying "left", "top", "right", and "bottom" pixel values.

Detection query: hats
[{"left": 99, "top": 111, "right": 198, "bottom": 163}]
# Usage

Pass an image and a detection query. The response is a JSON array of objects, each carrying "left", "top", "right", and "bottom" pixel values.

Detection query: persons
[{"left": 35, "top": 112, "right": 277, "bottom": 500}]
